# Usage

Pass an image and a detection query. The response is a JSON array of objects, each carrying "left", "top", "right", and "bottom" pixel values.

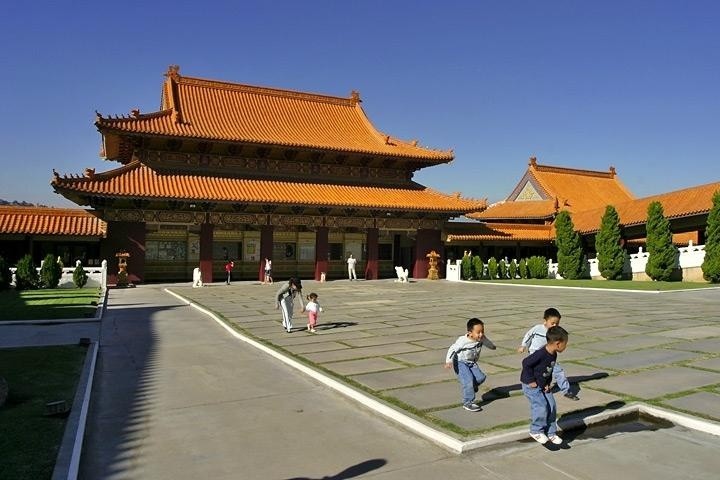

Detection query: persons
[
  {"left": 274, "top": 276, "right": 306, "bottom": 334},
  {"left": 516, "top": 306, "right": 580, "bottom": 403},
  {"left": 345, "top": 253, "right": 358, "bottom": 282},
  {"left": 223, "top": 259, "right": 235, "bottom": 285},
  {"left": 518, "top": 325, "right": 570, "bottom": 446},
  {"left": 299, "top": 291, "right": 325, "bottom": 334},
  {"left": 444, "top": 316, "right": 498, "bottom": 413},
  {"left": 191, "top": 263, "right": 204, "bottom": 289},
  {"left": 264, "top": 256, "right": 274, "bottom": 285}
]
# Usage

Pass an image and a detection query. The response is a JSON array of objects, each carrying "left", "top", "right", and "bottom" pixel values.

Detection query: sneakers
[
  {"left": 463, "top": 402, "right": 481, "bottom": 411},
  {"left": 547, "top": 434, "right": 563, "bottom": 445},
  {"left": 529, "top": 432, "right": 549, "bottom": 445}
]
